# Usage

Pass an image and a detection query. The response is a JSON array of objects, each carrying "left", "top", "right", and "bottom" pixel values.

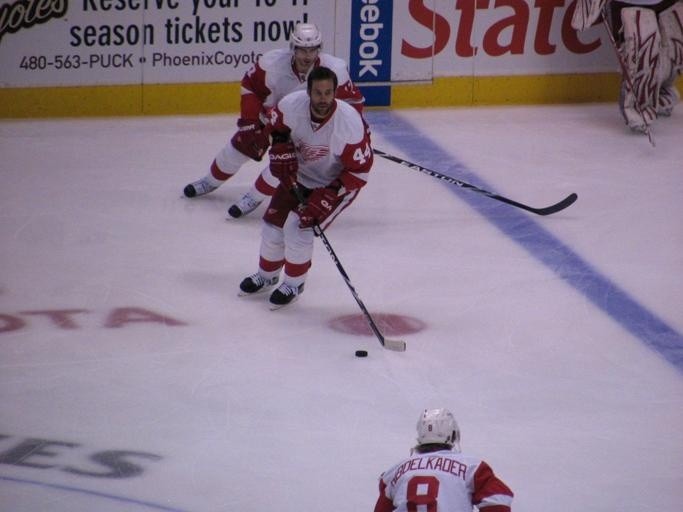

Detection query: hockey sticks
[
  {"left": 288, "top": 172, "right": 406, "bottom": 353},
  {"left": 600, "top": 10, "right": 657, "bottom": 148},
  {"left": 372, "top": 149, "right": 577, "bottom": 215}
]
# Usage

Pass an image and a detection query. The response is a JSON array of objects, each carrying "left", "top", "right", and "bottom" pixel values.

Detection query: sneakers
[
  {"left": 183, "top": 174, "right": 218, "bottom": 198},
  {"left": 237, "top": 270, "right": 280, "bottom": 294},
  {"left": 267, "top": 280, "right": 306, "bottom": 308},
  {"left": 227, "top": 190, "right": 262, "bottom": 219}
]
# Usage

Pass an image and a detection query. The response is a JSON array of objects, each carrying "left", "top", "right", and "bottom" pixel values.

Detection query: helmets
[
  {"left": 289, "top": 22, "right": 323, "bottom": 56},
  {"left": 416, "top": 407, "right": 463, "bottom": 451}
]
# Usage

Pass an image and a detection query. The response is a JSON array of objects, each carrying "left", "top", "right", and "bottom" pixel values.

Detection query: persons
[
  {"left": 240, "top": 66, "right": 374, "bottom": 308},
  {"left": 374, "top": 407, "right": 514, "bottom": 511},
  {"left": 570, "top": 0, "right": 682, "bottom": 135},
  {"left": 184, "top": 23, "right": 366, "bottom": 221}
]
